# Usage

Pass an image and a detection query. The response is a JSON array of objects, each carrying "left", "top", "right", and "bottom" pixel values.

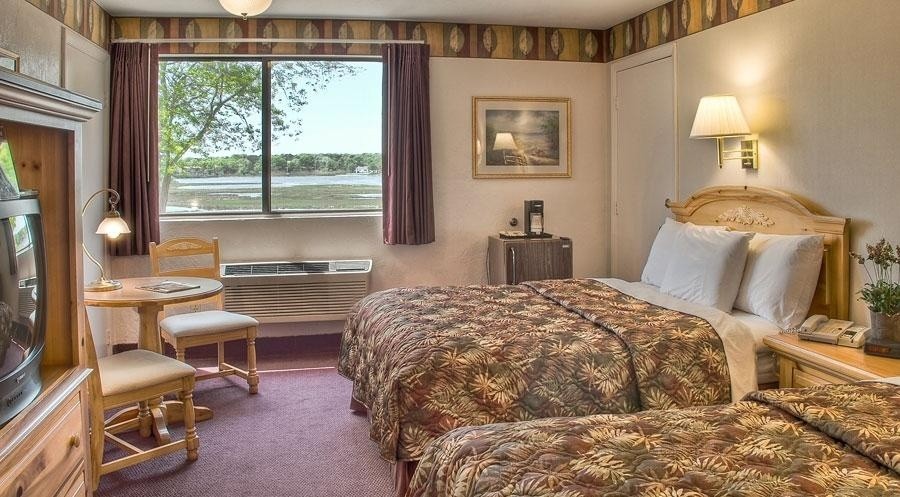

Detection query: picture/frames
[{"left": 470, "top": 94, "right": 574, "bottom": 180}]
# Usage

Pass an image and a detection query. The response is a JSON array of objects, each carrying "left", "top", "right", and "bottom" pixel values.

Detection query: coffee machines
[{"left": 523, "top": 199, "right": 551, "bottom": 238}]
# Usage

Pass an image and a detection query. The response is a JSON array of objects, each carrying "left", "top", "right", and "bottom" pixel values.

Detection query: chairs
[
  {"left": 82, "top": 303, "right": 201, "bottom": 493},
  {"left": 149, "top": 236, "right": 259, "bottom": 402}
]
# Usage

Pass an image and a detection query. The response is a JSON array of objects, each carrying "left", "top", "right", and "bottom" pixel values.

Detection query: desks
[{"left": 84, "top": 275, "right": 225, "bottom": 433}]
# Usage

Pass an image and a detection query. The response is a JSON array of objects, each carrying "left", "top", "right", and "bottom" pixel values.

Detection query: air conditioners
[{"left": 218, "top": 259, "right": 373, "bottom": 324}]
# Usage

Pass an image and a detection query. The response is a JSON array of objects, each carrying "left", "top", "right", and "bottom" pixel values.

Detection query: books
[{"left": 136, "top": 281, "right": 203, "bottom": 294}]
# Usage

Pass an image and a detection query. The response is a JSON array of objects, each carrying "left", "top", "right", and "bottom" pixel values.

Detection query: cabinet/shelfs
[{"left": 0, "top": 364, "right": 94, "bottom": 497}]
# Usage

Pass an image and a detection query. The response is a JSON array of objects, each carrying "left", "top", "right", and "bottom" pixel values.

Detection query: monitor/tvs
[{"left": 0, "top": 197, "right": 48, "bottom": 428}]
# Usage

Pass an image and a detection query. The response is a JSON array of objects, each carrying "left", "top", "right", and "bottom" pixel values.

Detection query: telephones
[{"left": 797, "top": 314, "right": 855, "bottom": 345}]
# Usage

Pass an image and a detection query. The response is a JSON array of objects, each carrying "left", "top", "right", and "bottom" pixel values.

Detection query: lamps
[
  {"left": 686, "top": 94, "right": 759, "bottom": 171},
  {"left": 80, "top": 189, "right": 132, "bottom": 293}
]
